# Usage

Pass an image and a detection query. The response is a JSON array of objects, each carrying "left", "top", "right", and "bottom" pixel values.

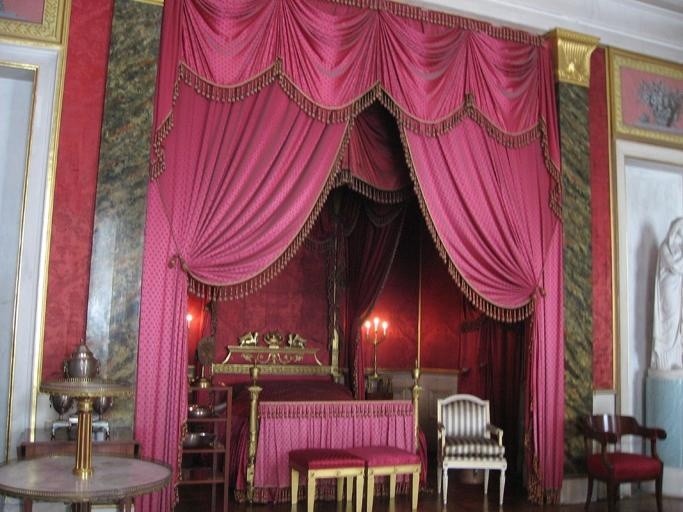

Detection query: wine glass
[
  {"left": 51, "top": 393, "right": 71, "bottom": 421},
  {"left": 93, "top": 396, "right": 113, "bottom": 422}
]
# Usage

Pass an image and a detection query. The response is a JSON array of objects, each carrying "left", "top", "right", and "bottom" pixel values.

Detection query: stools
[{"left": 285, "top": 445, "right": 422, "bottom": 512}]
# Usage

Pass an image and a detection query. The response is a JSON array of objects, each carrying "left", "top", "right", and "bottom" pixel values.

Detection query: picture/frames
[
  {"left": 0, "top": 2, "right": 71, "bottom": 49},
  {"left": 608, "top": 44, "right": 682, "bottom": 149}
]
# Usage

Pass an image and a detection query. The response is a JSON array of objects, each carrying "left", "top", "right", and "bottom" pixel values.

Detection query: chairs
[
  {"left": 577, "top": 414, "right": 670, "bottom": 512},
  {"left": 435, "top": 394, "right": 510, "bottom": 508}
]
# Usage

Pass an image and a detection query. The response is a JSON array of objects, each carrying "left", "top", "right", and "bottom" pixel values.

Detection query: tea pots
[{"left": 192, "top": 375, "right": 212, "bottom": 389}]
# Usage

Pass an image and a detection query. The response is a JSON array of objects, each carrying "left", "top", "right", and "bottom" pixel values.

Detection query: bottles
[
  {"left": 62, "top": 338, "right": 100, "bottom": 378},
  {"left": 190, "top": 406, "right": 211, "bottom": 419}
]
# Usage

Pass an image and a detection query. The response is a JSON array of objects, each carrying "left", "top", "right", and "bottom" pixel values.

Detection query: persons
[{"left": 650, "top": 217, "right": 683, "bottom": 370}]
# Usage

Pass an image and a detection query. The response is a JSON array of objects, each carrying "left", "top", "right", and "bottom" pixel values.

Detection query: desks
[
  {"left": 1, "top": 451, "right": 175, "bottom": 512},
  {"left": 20, "top": 428, "right": 134, "bottom": 512}
]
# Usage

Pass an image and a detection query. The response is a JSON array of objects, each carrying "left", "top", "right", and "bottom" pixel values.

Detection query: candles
[{"left": 365, "top": 322, "right": 389, "bottom": 336}]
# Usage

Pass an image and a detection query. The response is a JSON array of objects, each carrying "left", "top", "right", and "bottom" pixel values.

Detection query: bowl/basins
[{"left": 198, "top": 432, "right": 216, "bottom": 448}]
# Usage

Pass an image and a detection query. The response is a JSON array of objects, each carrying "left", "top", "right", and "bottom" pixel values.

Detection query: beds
[{"left": 216, "top": 332, "right": 428, "bottom": 507}]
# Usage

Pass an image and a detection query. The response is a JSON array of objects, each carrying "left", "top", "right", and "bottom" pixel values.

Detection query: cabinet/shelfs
[{"left": 183, "top": 382, "right": 233, "bottom": 512}]
